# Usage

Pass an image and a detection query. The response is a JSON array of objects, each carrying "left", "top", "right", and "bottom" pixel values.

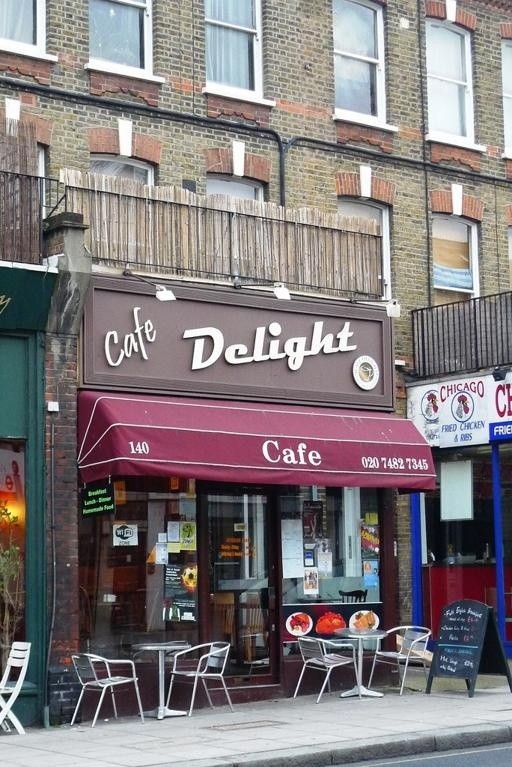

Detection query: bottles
[{"left": 484, "top": 543, "right": 491, "bottom": 561}]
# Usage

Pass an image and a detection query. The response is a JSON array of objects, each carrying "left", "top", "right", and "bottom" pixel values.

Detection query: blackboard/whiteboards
[{"left": 430, "top": 599, "right": 488, "bottom": 679}]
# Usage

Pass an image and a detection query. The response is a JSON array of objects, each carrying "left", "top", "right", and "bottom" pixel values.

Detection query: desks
[
  {"left": 297, "top": 596, "right": 343, "bottom": 604},
  {"left": 335, "top": 627, "right": 389, "bottom": 698},
  {"left": 130, "top": 642, "right": 190, "bottom": 720}
]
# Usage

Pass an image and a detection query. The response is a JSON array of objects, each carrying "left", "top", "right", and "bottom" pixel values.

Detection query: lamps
[
  {"left": 233, "top": 279, "right": 292, "bottom": 300},
  {"left": 123, "top": 269, "right": 176, "bottom": 302},
  {"left": 349, "top": 296, "right": 402, "bottom": 319}
]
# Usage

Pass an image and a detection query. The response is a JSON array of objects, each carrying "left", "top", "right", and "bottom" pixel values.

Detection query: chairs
[
  {"left": 209, "top": 592, "right": 269, "bottom": 663},
  {"left": 367, "top": 625, "right": 432, "bottom": 697},
  {"left": 166, "top": 641, "right": 235, "bottom": 717},
  {"left": 0, "top": 641, "right": 32, "bottom": 735},
  {"left": 293, "top": 635, "right": 364, "bottom": 704},
  {"left": 69, "top": 651, "right": 147, "bottom": 729},
  {"left": 337, "top": 589, "right": 369, "bottom": 603}
]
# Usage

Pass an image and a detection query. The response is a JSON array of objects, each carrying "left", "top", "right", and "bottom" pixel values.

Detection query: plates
[{"left": 284, "top": 610, "right": 379, "bottom": 638}]
